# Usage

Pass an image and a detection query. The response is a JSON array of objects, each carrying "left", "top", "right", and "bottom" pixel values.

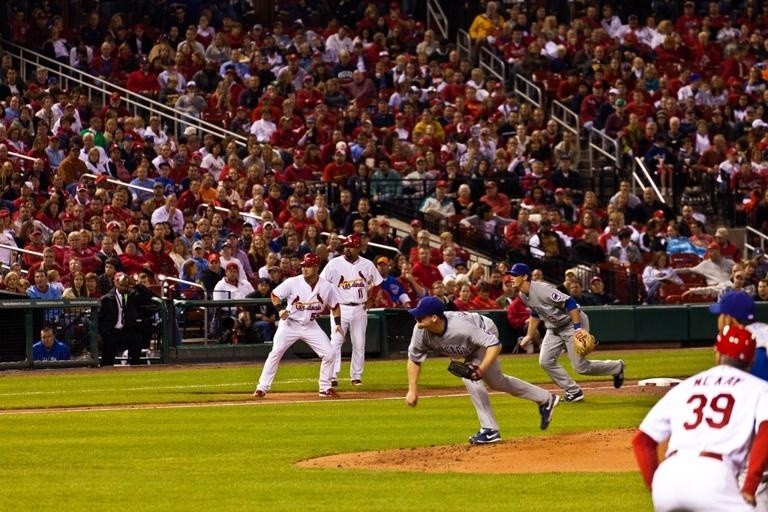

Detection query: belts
[
  {"left": 344, "top": 302, "right": 358, "bottom": 306},
  {"left": 665, "top": 449, "right": 721, "bottom": 460}
]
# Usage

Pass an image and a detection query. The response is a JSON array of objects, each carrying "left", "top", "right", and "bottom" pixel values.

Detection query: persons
[
  {"left": 706, "top": 291, "right": 768, "bottom": 512},
  {"left": 402, "top": 294, "right": 562, "bottom": 447},
  {"left": 0, "top": 1, "right": 767, "bottom": 370},
  {"left": 251, "top": 252, "right": 339, "bottom": 399},
  {"left": 629, "top": 325, "right": 768, "bottom": 512},
  {"left": 502, "top": 262, "right": 626, "bottom": 404},
  {"left": 318, "top": 232, "right": 384, "bottom": 387}
]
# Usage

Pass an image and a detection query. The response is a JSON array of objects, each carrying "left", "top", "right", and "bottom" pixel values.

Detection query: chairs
[{"left": 600, "top": 253, "right": 710, "bottom": 306}]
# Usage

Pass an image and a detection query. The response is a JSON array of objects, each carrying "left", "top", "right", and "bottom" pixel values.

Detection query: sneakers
[
  {"left": 613, "top": 360, "right": 624, "bottom": 388},
  {"left": 539, "top": 394, "right": 559, "bottom": 429},
  {"left": 560, "top": 390, "right": 584, "bottom": 402},
  {"left": 469, "top": 427, "right": 501, "bottom": 444},
  {"left": 254, "top": 377, "right": 362, "bottom": 398}
]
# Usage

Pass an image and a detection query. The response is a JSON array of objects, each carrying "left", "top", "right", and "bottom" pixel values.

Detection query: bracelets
[
  {"left": 334, "top": 316, "right": 341, "bottom": 325},
  {"left": 273, "top": 303, "right": 285, "bottom": 312},
  {"left": 572, "top": 321, "right": 583, "bottom": 331}
]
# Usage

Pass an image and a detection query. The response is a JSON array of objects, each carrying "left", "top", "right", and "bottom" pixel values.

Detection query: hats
[
  {"left": 709, "top": 291, "right": 757, "bottom": 320},
  {"left": 0, "top": 3, "right": 764, "bottom": 278},
  {"left": 408, "top": 297, "right": 444, "bottom": 316}
]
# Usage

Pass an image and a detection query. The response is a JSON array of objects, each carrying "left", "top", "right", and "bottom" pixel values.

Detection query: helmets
[{"left": 716, "top": 325, "right": 757, "bottom": 362}]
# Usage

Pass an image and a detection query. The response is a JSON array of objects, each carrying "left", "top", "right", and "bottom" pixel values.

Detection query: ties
[{"left": 121, "top": 293, "right": 127, "bottom": 325}]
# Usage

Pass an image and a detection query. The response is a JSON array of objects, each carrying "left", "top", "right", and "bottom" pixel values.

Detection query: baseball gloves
[
  {"left": 447, "top": 361, "right": 482, "bottom": 381},
  {"left": 574, "top": 328, "right": 596, "bottom": 356}
]
